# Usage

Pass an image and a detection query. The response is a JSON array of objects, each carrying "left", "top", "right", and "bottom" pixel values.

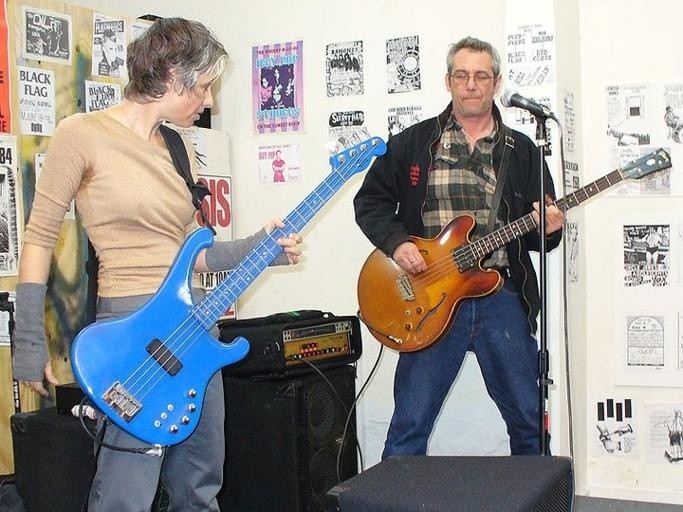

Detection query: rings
[{"left": 411, "top": 258, "right": 417, "bottom": 263}]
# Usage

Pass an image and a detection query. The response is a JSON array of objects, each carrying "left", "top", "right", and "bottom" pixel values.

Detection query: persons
[
  {"left": 328, "top": 53, "right": 360, "bottom": 72},
  {"left": 47, "top": 23, "right": 63, "bottom": 57},
  {"left": 664, "top": 106, "right": 683, "bottom": 144},
  {"left": 271, "top": 150, "right": 286, "bottom": 182},
  {"left": 351, "top": 36, "right": 565, "bottom": 463},
  {"left": 388, "top": 116, "right": 399, "bottom": 140},
  {"left": 11, "top": 17, "right": 303, "bottom": 510},
  {"left": 631, "top": 226, "right": 663, "bottom": 265},
  {"left": 260, "top": 64, "right": 294, "bottom": 110},
  {"left": 98, "top": 30, "right": 125, "bottom": 78}
]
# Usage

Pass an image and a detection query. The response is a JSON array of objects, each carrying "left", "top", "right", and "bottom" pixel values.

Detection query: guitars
[
  {"left": 358, "top": 147, "right": 672, "bottom": 353},
  {"left": 71, "top": 136, "right": 388, "bottom": 449}
]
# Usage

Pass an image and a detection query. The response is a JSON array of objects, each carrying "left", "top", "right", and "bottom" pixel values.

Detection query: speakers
[
  {"left": 215, "top": 365, "right": 358, "bottom": 512},
  {"left": 10, "top": 406, "right": 170, "bottom": 511}
]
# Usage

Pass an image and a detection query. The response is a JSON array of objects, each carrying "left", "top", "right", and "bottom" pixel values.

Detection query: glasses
[{"left": 449, "top": 69, "right": 496, "bottom": 86}]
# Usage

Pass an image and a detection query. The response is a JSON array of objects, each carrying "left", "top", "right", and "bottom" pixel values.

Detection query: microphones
[{"left": 497, "top": 84, "right": 545, "bottom": 119}]
